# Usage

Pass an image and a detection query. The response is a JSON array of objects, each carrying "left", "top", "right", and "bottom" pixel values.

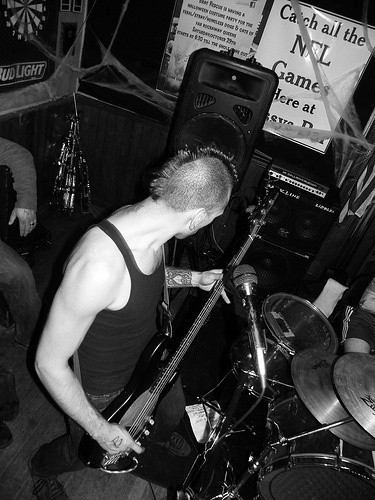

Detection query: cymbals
[
  {"left": 331, "top": 352, "right": 375, "bottom": 438},
  {"left": 290, "top": 349, "right": 375, "bottom": 451}
]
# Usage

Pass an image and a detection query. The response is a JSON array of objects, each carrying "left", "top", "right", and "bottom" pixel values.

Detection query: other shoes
[
  {"left": 30, "top": 457, "right": 69, "bottom": 500},
  {"left": 0, "top": 373, "right": 18, "bottom": 448},
  {"left": 101, "top": 454, "right": 140, "bottom": 473}
]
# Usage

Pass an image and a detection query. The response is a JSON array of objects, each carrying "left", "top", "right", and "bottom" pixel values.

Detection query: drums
[
  {"left": 230, "top": 290, "right": 340, "bottom": 399},
  {"left": 255, "top": 392, "right": 374, "bottom": 500}
]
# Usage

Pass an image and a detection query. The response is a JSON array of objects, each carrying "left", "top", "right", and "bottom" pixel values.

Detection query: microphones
[{"left": 232, "top": 264, "right": 267, "bottom": 388}]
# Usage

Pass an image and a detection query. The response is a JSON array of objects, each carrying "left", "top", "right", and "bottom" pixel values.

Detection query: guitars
[{"left": 79, "top": 171, "right": 283, "bottom": 466}]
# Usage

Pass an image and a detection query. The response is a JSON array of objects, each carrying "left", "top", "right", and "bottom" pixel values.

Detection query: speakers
[
  {"left": 160, "top": 48, "right": 279, "bottom": 193},
  {"left": 250, "top": 159, "right": 341, "bottom": 262}
]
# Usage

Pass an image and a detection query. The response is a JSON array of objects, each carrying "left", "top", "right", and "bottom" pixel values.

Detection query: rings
[{"left": 30, "top": 223, "right": 34, "bottom": 226}]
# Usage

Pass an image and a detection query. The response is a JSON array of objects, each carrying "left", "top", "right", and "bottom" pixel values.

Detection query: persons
[
  {"left": 339, "top": 269, "right": 375, "bottom": 355},
  {"left": 0, "top": 138, "right": 51, "bottom": 450},
  {"left": 27, "top": 148, "right": 232, "bottom": 500}
]
